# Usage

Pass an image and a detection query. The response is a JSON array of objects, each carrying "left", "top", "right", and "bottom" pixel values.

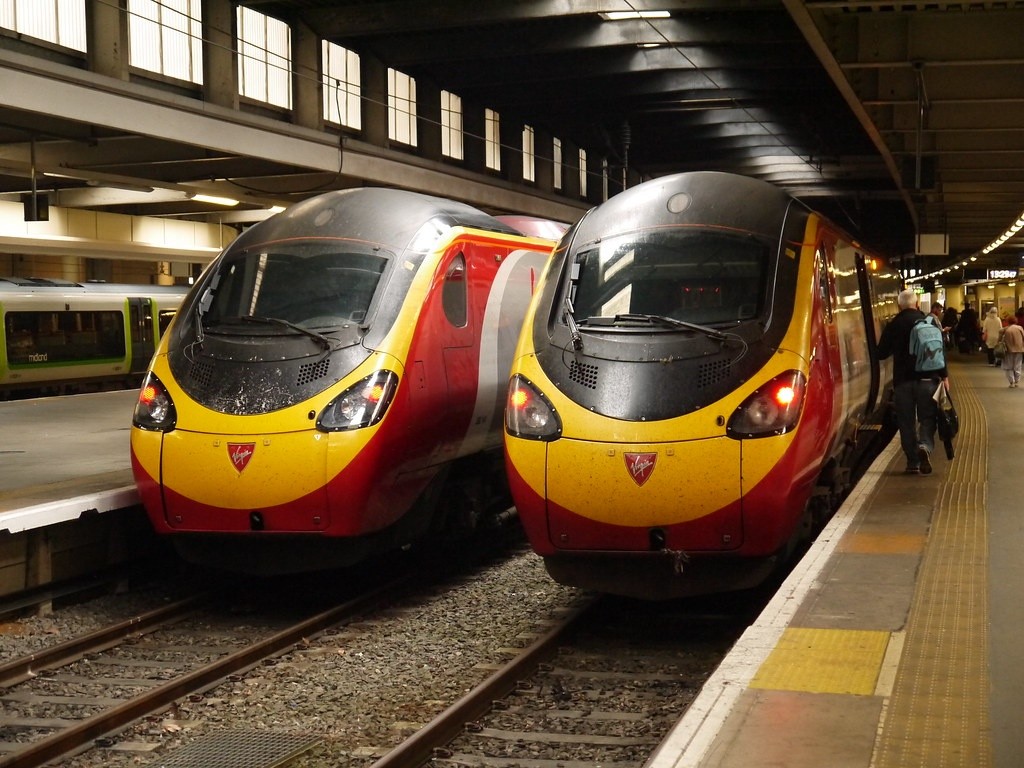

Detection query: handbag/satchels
[
  {"left": 994, "top": 341, "right": 1006, "bottom": 356},
  {"left": 982, "top": 334, "right": 986, "bottom": 340},
  {"left": 938, "top": 382, "right": 959, "bottom": 461}
]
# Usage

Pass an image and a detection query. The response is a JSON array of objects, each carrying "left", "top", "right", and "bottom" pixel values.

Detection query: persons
[
  {"left": 943, "top": 307, "right": 958, "bottom": 351},
  {"left": 1001, "top": 316, "right": 1024, "bottom": 388},
  {"left": 924, "top": 302, "right": 952, "bottom": 332},
  {"left": 1014, "top": 302, "right": 1024, "bottom": 326},
  {"left": 958, "top": 303, "right": 977, "bottom": 350},
  {"left": 981, "top": 306, "right": 1003, "bottom": 367},
  {"left": 874, "top": 289, "right": 950, "bottom": 476}
]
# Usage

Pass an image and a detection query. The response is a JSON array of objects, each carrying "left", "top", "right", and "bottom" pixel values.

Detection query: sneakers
[
  {"left": 917, "top": 442, "right": 933, "bottom": 474},
  {"left": 906, "top": 462, "right": 920, "bottom": 472}
]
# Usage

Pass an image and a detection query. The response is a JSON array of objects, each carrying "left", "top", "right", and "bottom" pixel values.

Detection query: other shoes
[
  {"left": 1009, "top": 383, "right": 1019, "bottom": 387},
  {"left": 990, "top": 363, "right": 996, "bottom": 366}
]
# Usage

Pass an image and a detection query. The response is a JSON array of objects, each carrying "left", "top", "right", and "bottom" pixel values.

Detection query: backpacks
[{"left": 910, "top": 316, "right": 948, "bottom": 377}]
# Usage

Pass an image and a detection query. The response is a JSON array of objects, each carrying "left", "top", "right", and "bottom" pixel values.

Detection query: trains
[
  {"left": 500, "top": 171, "right": 908, "bottom": 604},
  {"left": 0, "top": 276, "right": 193, "bottom": 402},
  {"left": 128, "top": 189, "right": 576, "bottom": 581}
]
